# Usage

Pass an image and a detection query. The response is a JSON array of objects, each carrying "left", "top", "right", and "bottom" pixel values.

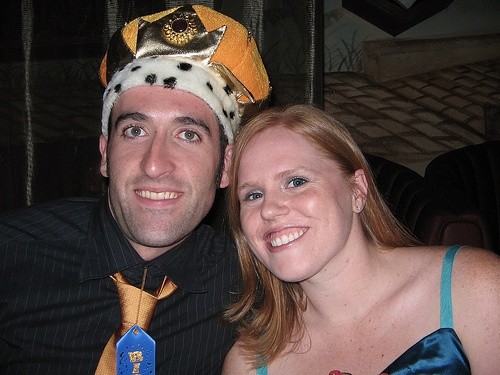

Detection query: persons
[
  {"left": 220, "top": 105, "right": 500, "bottom": 375},
  {"left": 0, "top": 3, "right": 270, "bottom": 375}
]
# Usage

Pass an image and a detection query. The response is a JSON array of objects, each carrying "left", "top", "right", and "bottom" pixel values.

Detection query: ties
[{"left": 94, "top": 273, "right": 178, "bottom": 375}]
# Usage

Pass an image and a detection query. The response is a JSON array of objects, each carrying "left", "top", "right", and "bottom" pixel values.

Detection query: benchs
[{"left": 361, "top": 140, "right": 500, "bottom": 253}]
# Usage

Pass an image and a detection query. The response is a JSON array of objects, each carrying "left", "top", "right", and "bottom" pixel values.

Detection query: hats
[{"left": 100, "top": 5, "right": 272, "bottom": 145}]
